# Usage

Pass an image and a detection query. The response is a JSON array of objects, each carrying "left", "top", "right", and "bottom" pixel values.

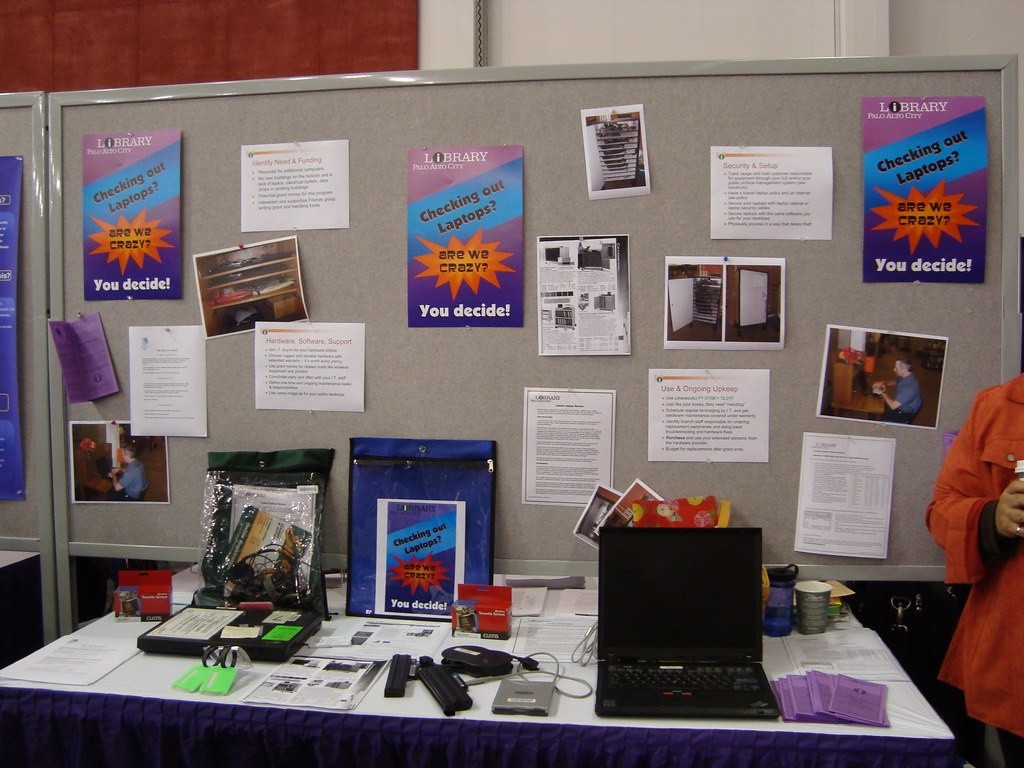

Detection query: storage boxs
[
  {"left": 452, "top": 583, "right": 513, "bottom": 641},
  {"left": 113, "top": 570, "right": 173, "bottom": 623}
]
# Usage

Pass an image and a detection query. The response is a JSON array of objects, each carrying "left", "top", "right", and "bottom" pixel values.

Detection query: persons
[
  {"left": 108, "top": 444, "right": 146, "bottom": 502},
  {"left": 872, "top": 355, "right": 920, "bottom": 423},
  {"left": 925, "top": 375, "right": 1024, "bottom": 768}
]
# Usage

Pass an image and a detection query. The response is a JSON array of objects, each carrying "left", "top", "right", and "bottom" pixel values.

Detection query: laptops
[
  {"left": 858, "top": 372, "right": 882, "bottom": 398},
  {"left": 96, "top": 456, "right": 123, "bottom": 481},
  {"left": 594, "top": 527, "right": 780, "bottom": 719}
]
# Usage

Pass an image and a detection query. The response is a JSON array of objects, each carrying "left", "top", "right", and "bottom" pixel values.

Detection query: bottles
[{"left": 762, "top": 563, "right": 799, "bottom": 637}]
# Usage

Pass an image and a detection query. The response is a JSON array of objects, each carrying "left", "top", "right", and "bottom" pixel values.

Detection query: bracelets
[
  {"left": 111, "top": 474, "right": 113, "bottom": 478},
  {"left": 881, "top": 392, "right": 885, "bottom": 397}
]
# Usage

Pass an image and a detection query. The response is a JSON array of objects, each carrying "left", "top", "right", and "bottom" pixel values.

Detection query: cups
[{"left": 794, "top": 581, "right": 834, "bottom": 635}]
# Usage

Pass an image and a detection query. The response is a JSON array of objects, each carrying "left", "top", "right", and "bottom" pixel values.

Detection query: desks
[{"left": 0, "top": 564, "right": 963, "bottom": 768}]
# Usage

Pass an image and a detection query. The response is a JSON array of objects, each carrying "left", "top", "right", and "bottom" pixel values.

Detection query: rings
[{"left": 1016, "top": 526, "right": 1020, "bottom": 536}]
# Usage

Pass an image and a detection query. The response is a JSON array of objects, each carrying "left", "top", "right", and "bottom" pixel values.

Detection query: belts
[{"left": 897, "top": 409, "right": 914, "bottom": 419}]
